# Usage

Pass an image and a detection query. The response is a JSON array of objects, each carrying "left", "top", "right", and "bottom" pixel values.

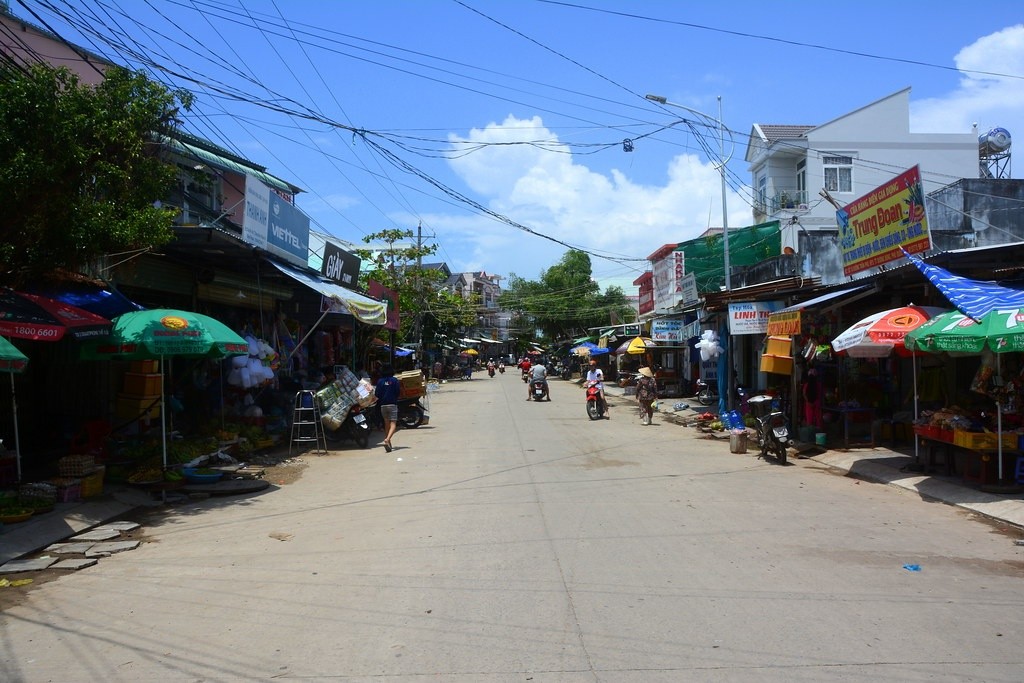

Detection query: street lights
[{"left": 644, "top": 93, "right": 736, "bottom": 411}]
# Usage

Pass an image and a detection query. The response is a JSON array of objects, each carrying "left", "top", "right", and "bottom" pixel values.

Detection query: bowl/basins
[
  {"left": 0, "top": 507, "right": 35, "bottom": 522},
  {"left": 183, "top": 469, "right": 223, "bottom": 484},
  {"left": 0, "top": 492, "right": 16, "bottom": 506}
]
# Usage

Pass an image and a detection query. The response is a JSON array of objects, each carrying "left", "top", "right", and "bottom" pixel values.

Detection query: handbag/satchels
[{"left": 650, "top": 399, "right": 657, "bottom": 410}]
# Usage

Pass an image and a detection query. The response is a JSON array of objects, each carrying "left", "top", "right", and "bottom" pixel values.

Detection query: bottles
[
  {"left": 720, "top": 411, "right": 732, "bottom": 429},
  {"left": 730, "top": 410, "right": 746, "bottom": 429}
]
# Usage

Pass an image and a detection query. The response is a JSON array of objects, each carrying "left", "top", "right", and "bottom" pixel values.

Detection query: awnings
[
  {"left": 262, "top": 258, "right": 388, "bottom": 325},
  {"left": 767, "top": 283, "right": 876, "bottom": 335},
  {"left": 439, "top": 337, "right": 503, "bottom": 349}
]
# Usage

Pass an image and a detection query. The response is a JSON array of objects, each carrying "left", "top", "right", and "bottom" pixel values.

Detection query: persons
[
  {"left": 166, "top": 357, "right": 193, "bottom": 411},
  {"left": 904, "top": 359, "right": 949, "bottom": 447},
  {"left": 636, "top": 367, "right": 658, "bottom": 425},
  {"left": 487, "top": 356, "right": 505, "bottom": 375},
  {"left": 583, "top": 360, "right": 610, "bottom": 417},
  {"left": 519, "top": 356, "right": 552, "bottom": 401},
  {"left": 802, "top": 369, "right": 825, "bottom": 434},
  {"left": 375, "top": 363, "right": 401, "bottom": 452}
]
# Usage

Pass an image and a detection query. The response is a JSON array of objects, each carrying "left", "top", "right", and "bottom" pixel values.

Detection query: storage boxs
[
  {"left": 912, "top": 424, "right": 1019, "bottom": 451},
  {"left": 59, "top": 471, "right": 104, "bottom": 502},
  {"left": 393, "top": 369, "right": 423, "bottom": 399},
  {"left": 760, "top": 335, "right": 793, "bottom": 375},
  {"left": 118, "top": 360, "right": 165, "bottom": 418},
  {"left": 625, "top": 386, "right": 636, "bottom": 395}
]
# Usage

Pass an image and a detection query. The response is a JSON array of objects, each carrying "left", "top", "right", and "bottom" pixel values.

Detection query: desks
[
  {"left": 824, "top": 405, "right": 877, "bottom": 449},
  {"left": 917, "top": 435, "right": 1024, "bottom": 490}
]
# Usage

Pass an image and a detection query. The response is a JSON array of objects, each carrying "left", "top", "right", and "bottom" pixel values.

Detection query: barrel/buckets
[{"left": 816, "top": 433, "right": 827, "bottom": 445}]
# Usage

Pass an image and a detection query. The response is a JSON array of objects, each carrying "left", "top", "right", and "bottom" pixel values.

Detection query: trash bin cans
[{"left": 730, "top": 433, "right": 748, "bottom": 454}]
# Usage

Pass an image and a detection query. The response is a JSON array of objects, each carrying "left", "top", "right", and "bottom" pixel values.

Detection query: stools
[
  {"left": 882, "top": 419, "right": 908, "bottom": 448},
  {"left": 1008, "top": 456, "right": 1024, "bottom": 488}
]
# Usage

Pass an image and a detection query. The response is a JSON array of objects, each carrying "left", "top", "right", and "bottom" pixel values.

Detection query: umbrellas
[
  {"left": 717, "top": 318, "right": 728, "bottom": 415},
  {"left": 461, "top": 349, "right": 478, "bottom": 354},
  {"left": 616, "top": 337, "right": 658, "bottom": 366},
  {"left": 831, "top": 303, "right": 956, "bottom": 460},
  {"left": 904, "top": 308, "right": 1024, "bottom": 480},
  {"left": 0, "top": 279, "right": 149, "bottom": 482},
  {"left": 529, "top": 350, "right": 541, "bottom": 355},
  {"left": 570, "top": 343, "right": 615, "bottom": 370},
  {"left": 80, "top": 307, "right": 250, "bottom": 472}
]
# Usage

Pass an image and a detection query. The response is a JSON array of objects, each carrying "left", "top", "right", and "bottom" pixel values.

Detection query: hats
[
  {"left": 808, "top": 369, "right": 818, "bottom": 377},
  {"left": 638, "top": 366, "right": 654, "bottom": 378}
]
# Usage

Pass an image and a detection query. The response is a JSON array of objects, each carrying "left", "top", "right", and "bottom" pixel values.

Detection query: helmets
[
  {"left": 524, "top": 357, "right": 528, "bottom": 361},
  {"left": 588, "top": 361, "right": 596, "bottom": 367},
  {"left": 500, "top": 357, "right": 503, "bottom": 359},
  {"left": 490, "top": 358, "right": 493, "bottom": 361}
]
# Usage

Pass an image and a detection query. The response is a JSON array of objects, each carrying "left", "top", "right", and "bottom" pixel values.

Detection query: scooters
[
  {"left": 370, "top": 396, "right": 429, "bottom": 432},
  {"left": 529, "top": 372, "right": 547, "bottom": 402},
  {"left": 543, "top": 356, "right": 581, "bottom": 381},
  {"left": 321, "top": 403, "right": 374, "bottom": 449},
  {"left": 498, "top": 362, "right": 505, "bottom": 374},
  {"left": 486, "top": 363, "right": 496, "bottom": 378},
  {"left": 521, "top": 367, "right": 530, "bottom": 383},
  {"left": 695, "top": 378, "right": 728, "bottom": 406},
  {"left": 583, "top": 373, "right": 609, "bottom": 420}
]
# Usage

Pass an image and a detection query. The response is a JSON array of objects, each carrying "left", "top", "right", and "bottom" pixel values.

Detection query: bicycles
[
  {"left": 459, "top": 361, "right": 472, "bottom": 382},
  {"left": 635, "top": 395, "right": 653, "bottom": 426}
]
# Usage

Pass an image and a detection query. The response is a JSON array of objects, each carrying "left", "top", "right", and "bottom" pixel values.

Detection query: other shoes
[
  {"left": 606, "top": 411, "right": 610, "bottom": 418},
  {"left": 548, "top": 398, "right": 551, "bottom": 401},
  {"left": 382, "top": 440, "right": 392, "bottom": 453},
  {"left": 642, "top": 419, "right": 652, "bottom": 425},
  {"left": 526, "top": 397, "right": 532, "bottom": 401}
]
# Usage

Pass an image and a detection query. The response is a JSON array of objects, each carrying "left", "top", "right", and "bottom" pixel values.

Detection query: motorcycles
[{"left": 738, "top": 387, "right": 795, "bottom": 465}]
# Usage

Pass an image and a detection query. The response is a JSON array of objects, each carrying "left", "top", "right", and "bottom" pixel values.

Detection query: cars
[{"left": 501, "top": 353, "right": 516, "bottom": 366}]
